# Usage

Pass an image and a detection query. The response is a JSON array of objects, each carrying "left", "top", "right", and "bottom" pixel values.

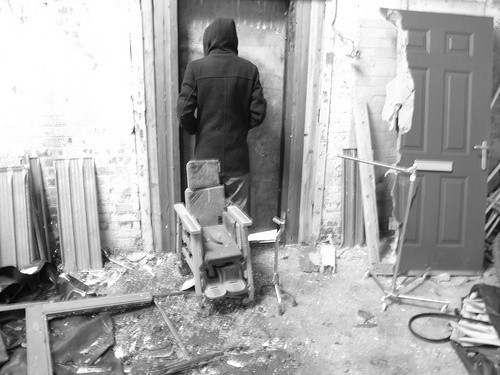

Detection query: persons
[{"left": 176, "top": 18, "right": 267, "bottom": 279}]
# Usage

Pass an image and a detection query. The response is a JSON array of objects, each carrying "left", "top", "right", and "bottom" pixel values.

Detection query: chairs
[{"left": 173, "top": 159, "right": 254, "bottom": 308}]
